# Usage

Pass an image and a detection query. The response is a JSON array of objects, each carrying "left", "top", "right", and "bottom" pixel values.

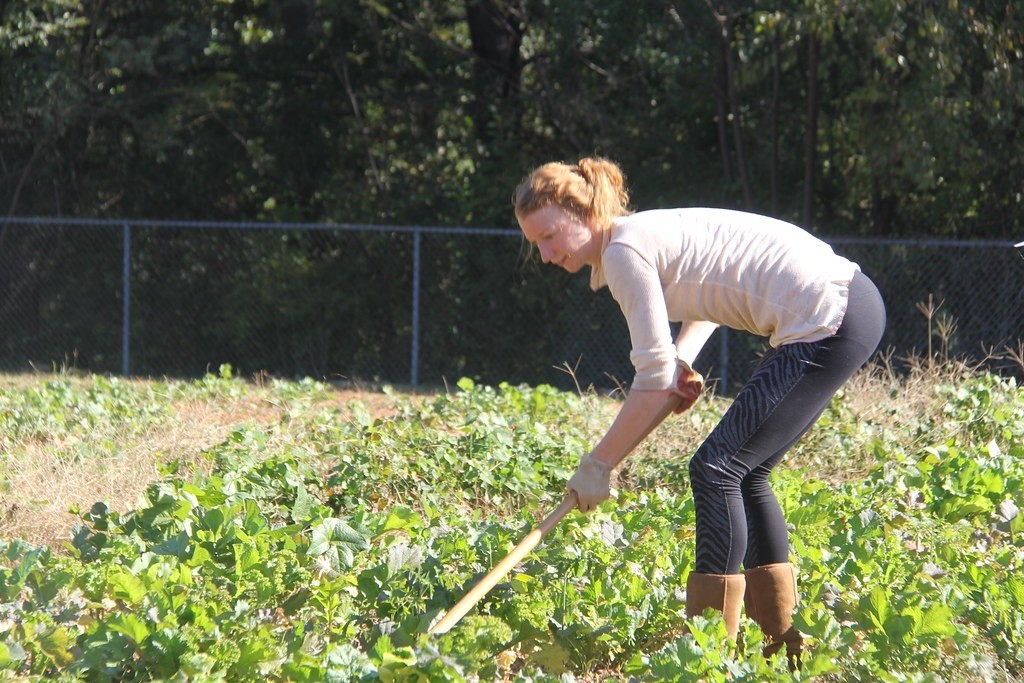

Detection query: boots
[
  {"left": 686, "top": 571, "right": 746, "bottom": 662},
  {"left": 744, "top": 563, "right": 802, "bottom": 674}
]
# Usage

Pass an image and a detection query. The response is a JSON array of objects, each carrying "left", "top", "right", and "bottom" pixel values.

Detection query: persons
[{"left": 514, "top": 158, "right": 887, "bottom": 671}]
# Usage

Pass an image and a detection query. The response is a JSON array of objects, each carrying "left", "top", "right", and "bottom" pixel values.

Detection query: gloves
[
  {"left": 565, "top": 455, "right": 614, "bottom": 514},
  {"left": 674, "top": 358, "right": 704, "bottom": 415}
]
